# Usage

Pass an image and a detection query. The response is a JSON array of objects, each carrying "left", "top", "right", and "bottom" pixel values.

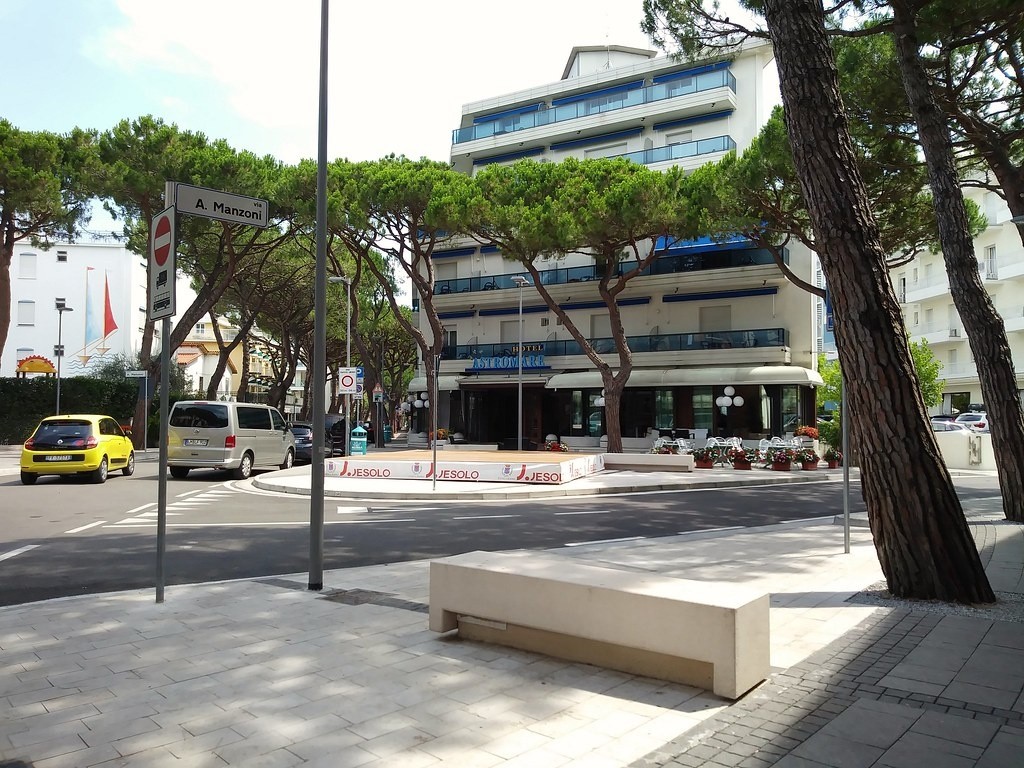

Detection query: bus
[{"left": 353, "top": 420, "right": 374, "bottom": 443}]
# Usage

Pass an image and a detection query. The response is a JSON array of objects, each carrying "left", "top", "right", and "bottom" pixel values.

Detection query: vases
[
  {"left": 829, "top": 460, "right": 839, "bottom": 469},
  {"left": 431, "top": 440, "right": 451, "bottom": 450},
  {"left": 734, "top": 461, "right": 751, "bottom": 470},
  {"left": 802, "top": 462, "right": 817, "bottom": 470},
  {"left": 696, "top": 460, "right": 713, "bottom": 468},
  {"left": 772, "top": 462, "right": 790, "bottom": 471},
  {"left": 801, "top": 437, "right": 819, "bottom": 450}
]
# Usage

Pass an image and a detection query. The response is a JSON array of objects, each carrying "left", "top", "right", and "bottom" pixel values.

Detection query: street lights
[
  {"left": 328, "top": 276, "right": 350, "bottom": 456},
  {"left": 511, "top": 275, "right": 531, "bottom": 452},
  {"left": 56, "top": 307, "right": 74, "bottom": 416}
]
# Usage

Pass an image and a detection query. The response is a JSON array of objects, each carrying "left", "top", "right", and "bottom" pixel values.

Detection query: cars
[
  {"left": 783, "top": 413, "right": 840, "bottom": 430},
  {"left": 931, "top": 420, "right": 975, "bottom": 434},
  {"left": 288, "top": 420, "right": 314, "bottom": 465},
  {"left": 929, "top": 413, "right": 958, "bottom": 424},
  {"left": 573, "top": 411, "right": 603, "bottom": 433},
  {"left": 950, "top": 412, "right": 990, "bottom": 434},
  {"left": 967, "top": 403, "right": 986, "bottom": 413},
  {"left": 20, "top": 414, "right": 135, "bottom": 485}
]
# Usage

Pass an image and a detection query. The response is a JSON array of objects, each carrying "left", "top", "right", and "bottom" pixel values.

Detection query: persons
[{"left": 100, "top": 422, "right": 106, "bottom": 433}]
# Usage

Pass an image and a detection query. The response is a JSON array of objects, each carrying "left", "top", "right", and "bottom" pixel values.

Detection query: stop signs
[{"left": 154, "top": 215, "right": 171, "bottom": 266}]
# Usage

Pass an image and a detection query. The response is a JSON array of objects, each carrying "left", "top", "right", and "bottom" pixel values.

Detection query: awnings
[
  {"left": 545, "top": 366, "right": 823, "bottom": 388},
  {"left": 407, "top": 376, "right": 467, "bottom": 391}
]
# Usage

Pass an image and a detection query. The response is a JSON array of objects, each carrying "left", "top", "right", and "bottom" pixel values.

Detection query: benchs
[{"left": 428, "top": 549, "right": 772, "bottom": 702}]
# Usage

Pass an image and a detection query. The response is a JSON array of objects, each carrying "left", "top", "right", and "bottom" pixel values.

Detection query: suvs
[{"left": 323, "top": 411, "right": 356, "bottom": 458}]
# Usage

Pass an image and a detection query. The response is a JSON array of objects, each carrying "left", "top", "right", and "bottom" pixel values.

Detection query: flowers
[
  {"left": 797, "top": 449, "right": 820, "bottom": 463},
  {"left": 769, "top": 447, "right": 797, "bottom": 464},
  {"left": 544, "top": 441, "right": 568, "bottom": 452},
  {"left": 655, "top": 447, "right": 677, "bottom": 454},
  {"left": 794, "top": 425, "right": 818, "bottom": 440},
  {"left": 730, "top": 448, "right": 760, "bottom": 464},
  {"left": 823, "top": 448, "right": 843, "bottom": 461},
  {"left": 693, "top": 448, "right": 720, "bottom": 460},
  {"left": 430, "top": 428, "right": 448, "bottom": 440}
]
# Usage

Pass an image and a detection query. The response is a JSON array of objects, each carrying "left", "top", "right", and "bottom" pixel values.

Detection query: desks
[
  {"left": 768, "top": 446, "right": 799, "bottom": 469},
  {"left": 713, "top": 445, "right": 734, "bottom": 467}
]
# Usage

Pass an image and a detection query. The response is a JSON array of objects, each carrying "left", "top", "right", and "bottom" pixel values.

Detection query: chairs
[
  {"left": 673, "top": 430, "right": 695, "bottom": 440},
  {"left": 652, "top": 436, "right": 802, "bottom": 468},
  {"left": 657, "top": 429, "right": 675, "bottom": 442}
]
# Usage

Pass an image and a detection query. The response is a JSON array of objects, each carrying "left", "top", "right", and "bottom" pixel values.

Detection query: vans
[{"left": 166, "top": 399, "right": 297, "bottom": 480}]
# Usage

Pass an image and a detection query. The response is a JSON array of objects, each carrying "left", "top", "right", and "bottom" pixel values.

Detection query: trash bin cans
[
  {"left": 350, "top": 425, "right": 368, "bottom": 456},
  {"left": 383, "top": 425, "right": 392, "bottom": 443}
]
